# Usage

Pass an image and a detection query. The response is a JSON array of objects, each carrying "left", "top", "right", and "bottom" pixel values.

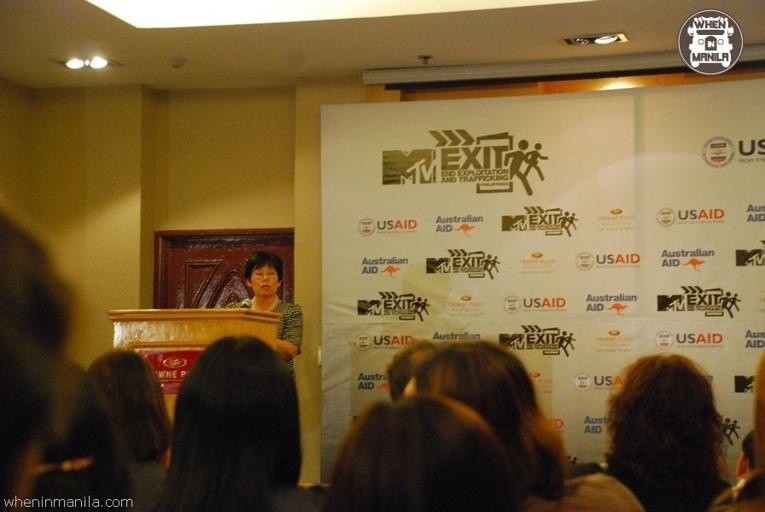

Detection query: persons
[
  {"left": 153, "top": 334, "right": 330, "bottom": 512},
  {"left": 319, "top": 339, "right": 764, "bottom": 511},
  {"left": 42, "top": 349, "right": 172, "bottom": 512},
  {"left": 0, "top": 209, "right": 78, "bottom": 512},
  {"left": 224, "top": 251, "right": 304, "bottom": 382}
]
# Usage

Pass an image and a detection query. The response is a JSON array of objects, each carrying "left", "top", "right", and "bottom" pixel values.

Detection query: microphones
[{"left": 240, "top": 296, "right": 253, "bottom": 309}]
